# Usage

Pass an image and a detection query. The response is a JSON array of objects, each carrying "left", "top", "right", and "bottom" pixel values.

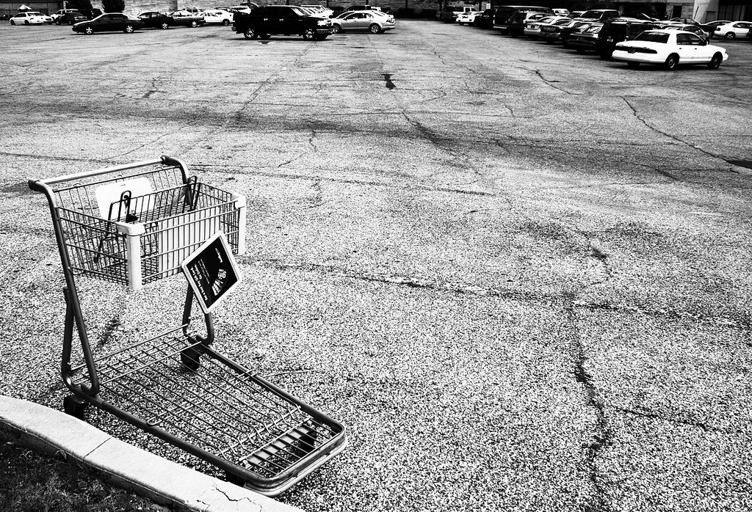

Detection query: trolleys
[{"left": 28, "top": 156, "right": 351, "bottom": 496}]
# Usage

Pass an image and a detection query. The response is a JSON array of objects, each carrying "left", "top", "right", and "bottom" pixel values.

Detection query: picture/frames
[{"left": 181, "top": 230, "right": 242, "bottom": 315}]
